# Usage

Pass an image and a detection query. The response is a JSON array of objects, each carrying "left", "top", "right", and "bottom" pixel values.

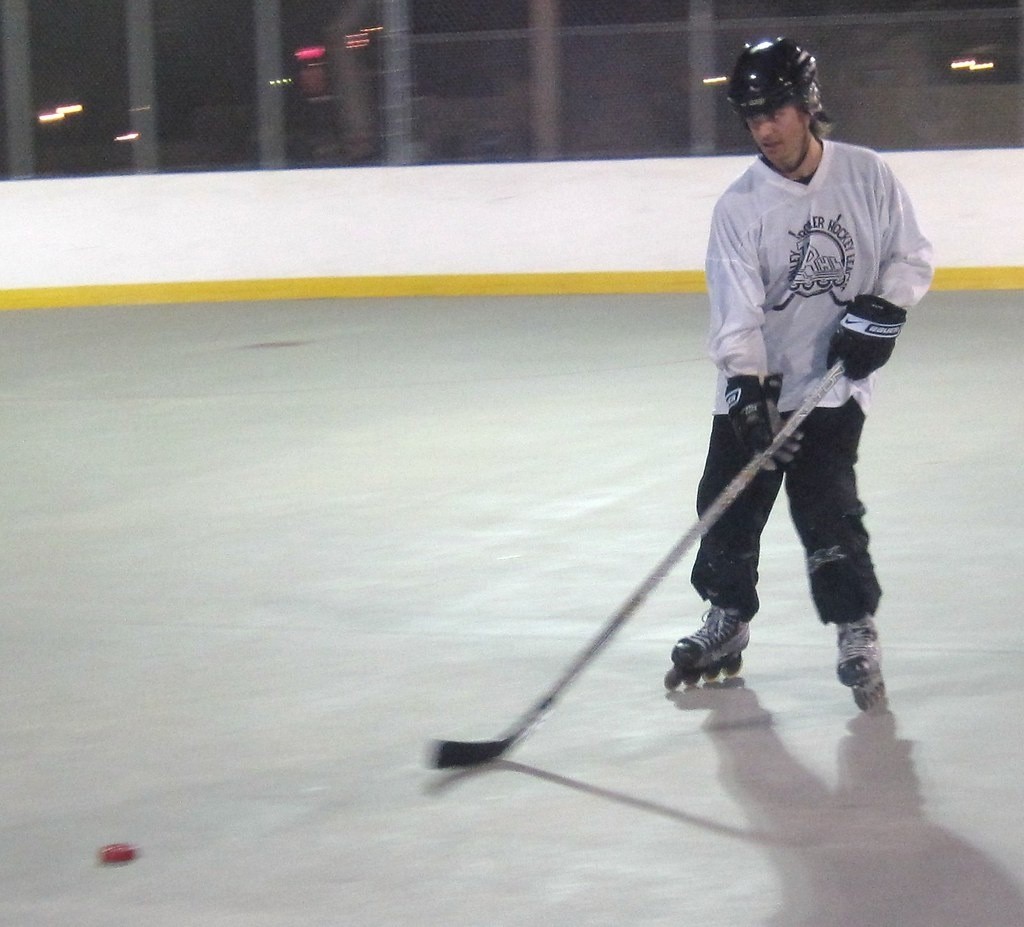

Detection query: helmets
[{"left": 724, "top": 36, "right": 822, "bottom": 118}]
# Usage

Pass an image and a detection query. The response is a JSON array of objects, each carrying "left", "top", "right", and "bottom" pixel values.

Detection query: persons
[{"left": 664, "top": 36, "right": 935, "bottom": 708}]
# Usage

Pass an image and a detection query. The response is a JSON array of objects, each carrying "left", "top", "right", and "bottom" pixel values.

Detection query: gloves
[
  {"left": 826, "top": 294, "right": 907, "bottom": 379},
  {"left": 725, "top": 372, "right": 804, "bottom": 471}
]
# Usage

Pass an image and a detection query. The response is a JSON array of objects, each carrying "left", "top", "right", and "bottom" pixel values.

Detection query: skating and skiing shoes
[
  {"left": 837, "top": 612, "right": 885, "bottom": 710},
  {"left": 664, "top": 605, "right": 750, "bottom": 694}
]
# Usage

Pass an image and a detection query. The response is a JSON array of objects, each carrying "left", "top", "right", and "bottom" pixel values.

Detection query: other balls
[{"left": 97, "top": 842, "right": 136, "bottom": 865}]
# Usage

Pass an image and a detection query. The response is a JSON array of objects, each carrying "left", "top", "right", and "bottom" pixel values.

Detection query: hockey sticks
[{"left": 412, "top": 353, "right": 846, "bottom": 773}]
